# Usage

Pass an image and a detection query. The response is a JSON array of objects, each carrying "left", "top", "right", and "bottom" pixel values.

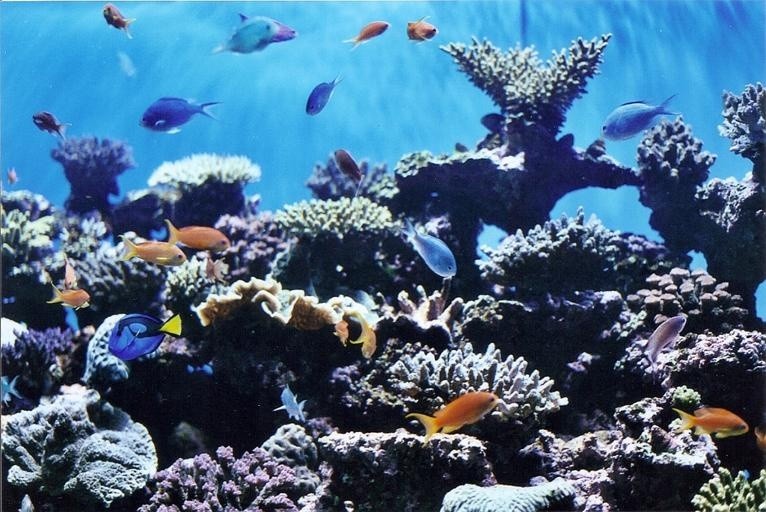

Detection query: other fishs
[{"left": 45, "top": 217, "right": 231, "bottom": 364}]
